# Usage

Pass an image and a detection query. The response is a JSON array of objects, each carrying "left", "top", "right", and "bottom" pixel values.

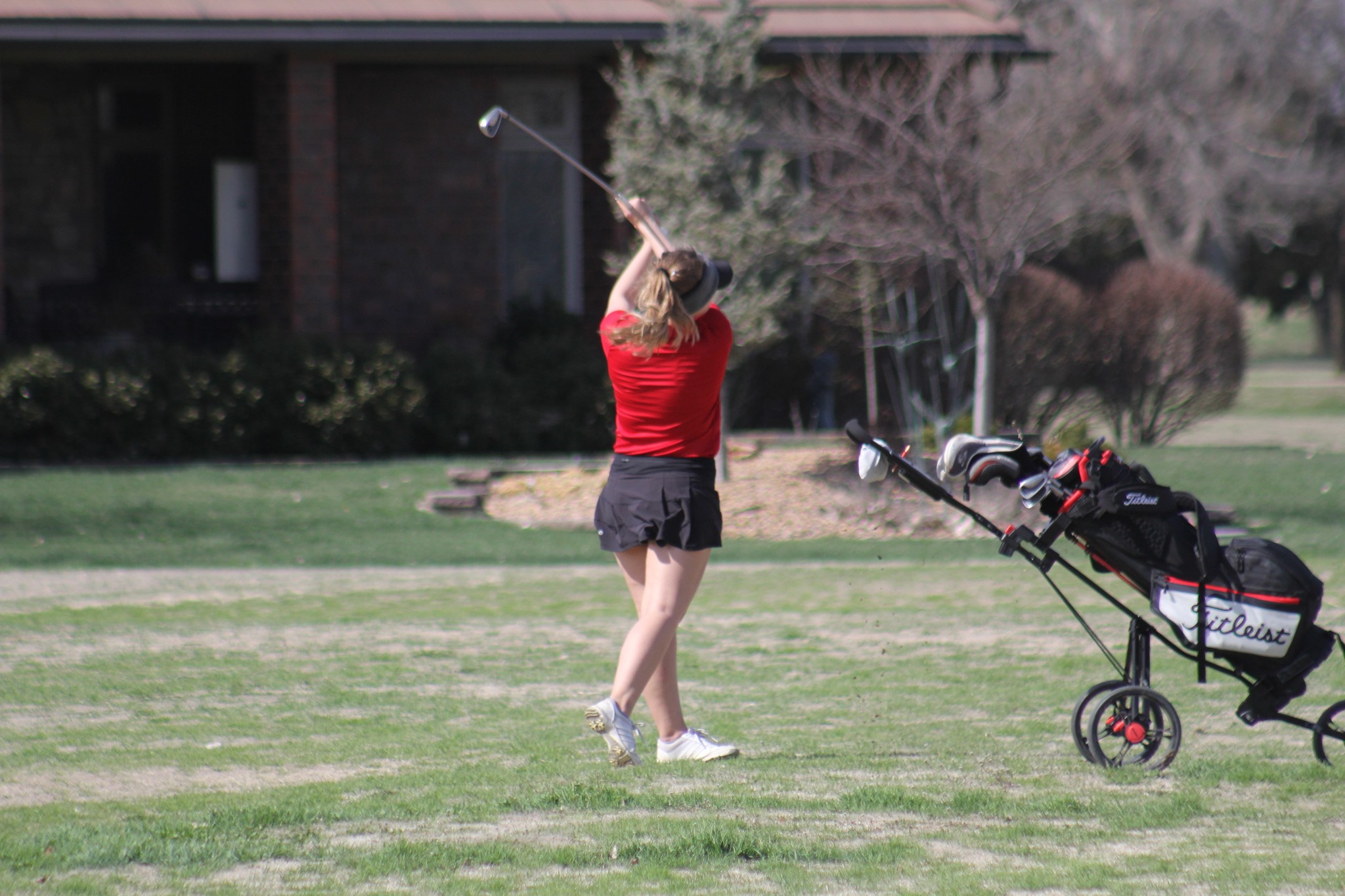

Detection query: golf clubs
[{"left": 471, "top": 105, "right": 634, "bottom": 212}]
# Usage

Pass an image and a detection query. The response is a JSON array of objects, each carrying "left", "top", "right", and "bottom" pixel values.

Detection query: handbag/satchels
[{"left": 1224, "top": 536, "right": 1324, "bottom": 650}]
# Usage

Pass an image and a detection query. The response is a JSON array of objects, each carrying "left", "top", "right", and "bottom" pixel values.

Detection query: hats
[{"left": 668, "top": 250, "right": 733, "bottom": 316}]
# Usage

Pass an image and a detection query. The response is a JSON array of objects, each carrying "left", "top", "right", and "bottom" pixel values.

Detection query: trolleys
[{"left": 839, "top": 419, "right": 1344, "bottom": 776}]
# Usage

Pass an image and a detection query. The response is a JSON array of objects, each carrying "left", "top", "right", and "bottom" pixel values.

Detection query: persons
[{"left": 582, "top": 198, "right": 740, "bottom": 765}]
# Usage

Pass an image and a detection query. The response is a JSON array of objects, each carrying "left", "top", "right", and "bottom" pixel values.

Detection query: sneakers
[
  {"left": 584, "top": 698, "right": 646, "bottom": 768},
  {"left": 657, "top": 728, "right": 739, "bottom": 764}
]
